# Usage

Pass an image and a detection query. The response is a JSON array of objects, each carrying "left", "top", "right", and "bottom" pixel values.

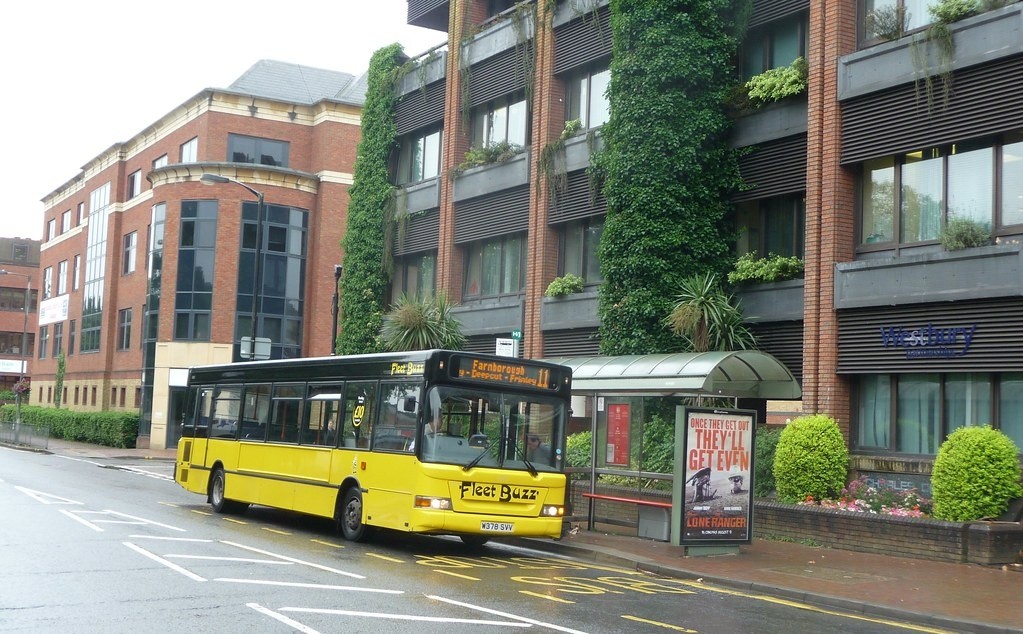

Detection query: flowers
[{"left": 11, "top": 379, "right": 32, "bottom": 398}]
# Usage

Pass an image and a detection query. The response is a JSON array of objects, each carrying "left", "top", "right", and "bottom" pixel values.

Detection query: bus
[{"left": 174, "top": 348, "right": 573, "bottom": 548}]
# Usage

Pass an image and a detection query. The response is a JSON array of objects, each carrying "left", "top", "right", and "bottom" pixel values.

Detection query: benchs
[{"left": 583, "top": 492, "right": 674, "bottom": 542}]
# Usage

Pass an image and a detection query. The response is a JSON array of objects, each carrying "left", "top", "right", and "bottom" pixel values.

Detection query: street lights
[
  {"left": 331, "top": 265, "right": 342, "bottom": 352},
  {"left": 200, "top": 173, "right": 264, "bottom": 361},
  {"left": 0, "top": 270, "right": 32, "bottom": 443}
]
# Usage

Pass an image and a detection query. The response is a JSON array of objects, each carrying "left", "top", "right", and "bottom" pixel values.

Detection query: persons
[
  {"left": 409, "top": 408, "right": 443, "bottom": 452},
  {"left": 527, "top": 432, "right": 552, "bottom": 465}
]
